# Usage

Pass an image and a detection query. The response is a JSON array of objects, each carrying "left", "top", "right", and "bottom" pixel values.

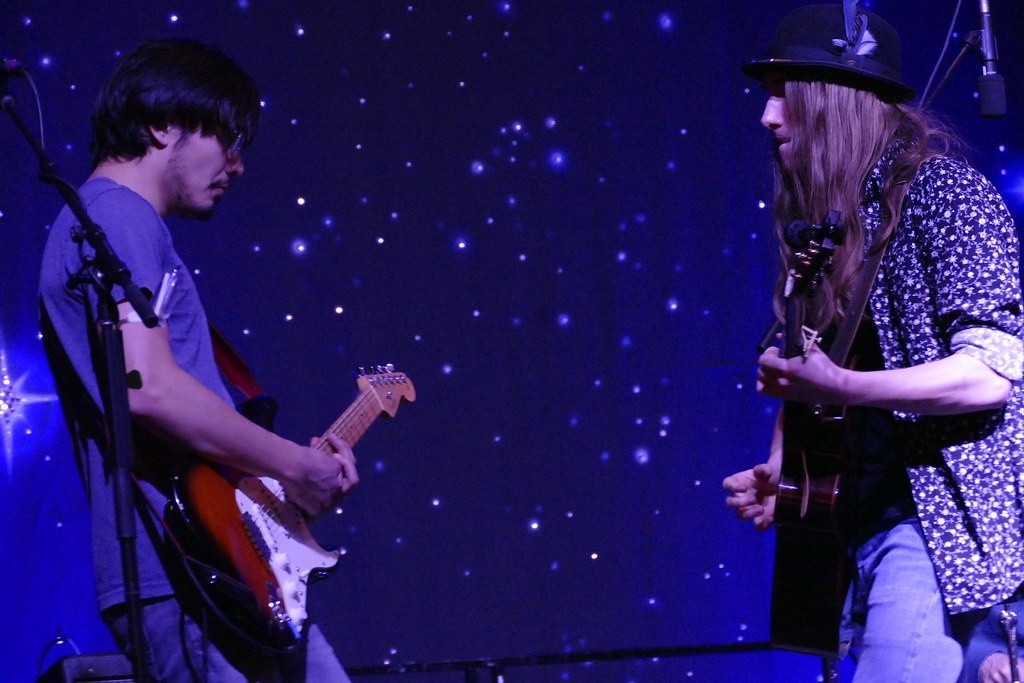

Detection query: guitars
[
  {"left": 162, "top": 359, "right": 417, "bottom": 661},
  {"left": 756, "top": 205, "right": 849, "bottom": 661}
]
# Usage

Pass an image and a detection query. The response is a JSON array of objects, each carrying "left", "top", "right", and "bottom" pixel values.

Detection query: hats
[{"left": 741, "top": 0, "right": 919, "bottom": 98}]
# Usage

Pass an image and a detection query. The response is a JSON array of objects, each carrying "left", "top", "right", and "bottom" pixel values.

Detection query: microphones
[
  {"left": 975, "top": 0, "right": 1007, "bottom": 118},
  {"left": 783, "top": 218, "right": 845, "bottom": 249},
  {"left": 0, "top": 57, "right": 30, "bottom": 81}
]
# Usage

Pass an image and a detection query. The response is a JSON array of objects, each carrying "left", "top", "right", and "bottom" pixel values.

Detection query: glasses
[{"left": 222, "top": 126, "right": 249, "bottom": 165}]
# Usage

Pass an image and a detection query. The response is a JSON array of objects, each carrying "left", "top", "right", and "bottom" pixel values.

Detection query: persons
[
  {"left": 964, "top": 598, "right": 1024, "bottom": 683},
  {"left": 36, "top": 37, "right": 360, "bottom": 683},
  {"left": 722, "top": 0, "right": 1024, "bottom": 683}
]
